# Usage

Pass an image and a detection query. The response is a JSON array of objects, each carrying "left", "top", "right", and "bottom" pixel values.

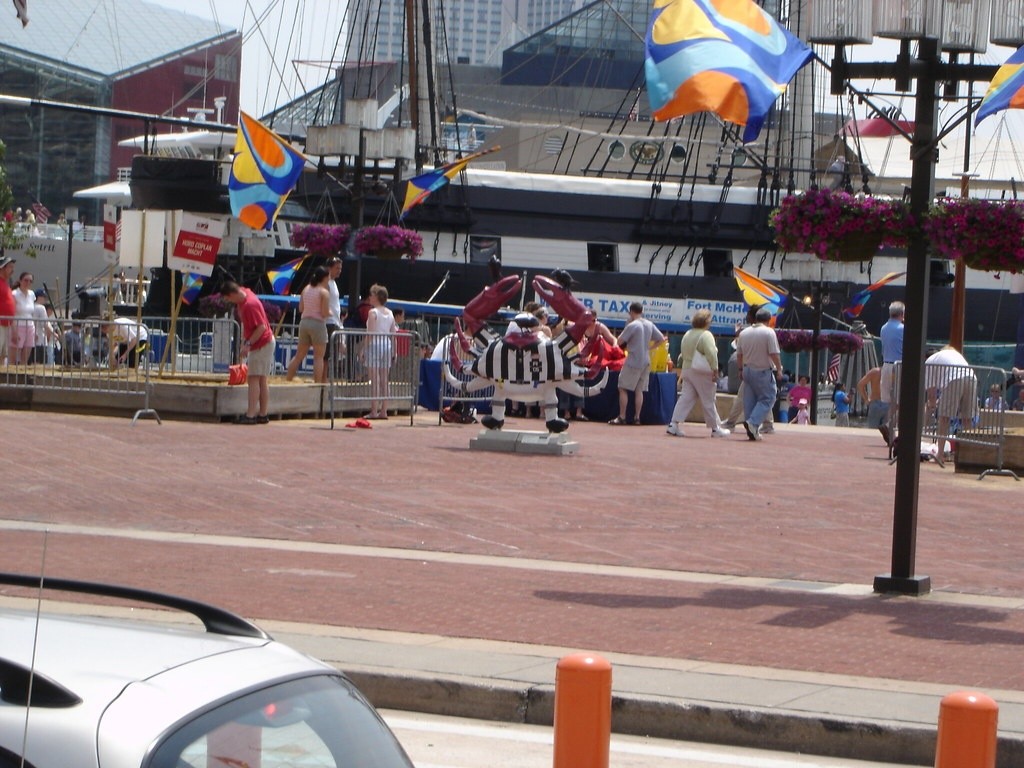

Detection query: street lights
[
  {"left": 63, "top": 206, "right": 79, "bottom": 318},
  {"left": 801, "top": 1, "right": 1024, "bottom": 594}
]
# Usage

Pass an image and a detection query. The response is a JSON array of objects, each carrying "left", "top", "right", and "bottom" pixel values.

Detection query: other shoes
[
  {"left": 929, "top": 455, "right": 945, "bottom": 463},
  {"left": 565, "top": 415, "right": 573, "bottom": 420},
  {"left": 721, "top": 420, "right": 735, "bottom": 432},
  {"left": 231, "top": 413, "right": 256, "bottom": 424},
  {"left": 575, "top": 415, "right": 589, "bottom": 421},
  {"left": 667, "top": 424, "right": 684, "bottom": 436},
  {"left": 744, "top": 420, "right": 762, "bottom": 440},
  {"left": 711, "top": 426, "right": 730, "bottom": 438},
  {"left": 945, "top": 454, "right": 955, "bottom": 461},
  {"left": 758, "top": 421, "right": 775, "bottom": 433},
  {"left": 255, "top": 414, "right": 270, "bottom": 423}
]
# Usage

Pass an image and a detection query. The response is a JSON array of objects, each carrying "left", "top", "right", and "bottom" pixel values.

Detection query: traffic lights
[{"left": 930, "top": 259, "right": 956, "bottom": 284}]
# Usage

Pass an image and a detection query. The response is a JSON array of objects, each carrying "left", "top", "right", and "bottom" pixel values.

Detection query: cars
[{"left": 1, "top": 572, "right": 419, "bottom": 768}]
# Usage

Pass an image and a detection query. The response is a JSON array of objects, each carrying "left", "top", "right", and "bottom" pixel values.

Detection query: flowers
[
  {"left": 768, "top": 186, "right": 919, "bottom": 255},
  {"left": 261, "top": 299, "right": 281, "bottom": 323},
  {"left": 776, "top": 330, "right": 864, "bottom": 356},
  {"left": 354, "top": 225, "right": 424, "bottom": 263},
  {"left": 197, "top": 294, "right": 236, "bottom": 315},
  {"left": 921, "top": 194, "right": 1024, "bottom": 279},
  {"left": 289, "top": 224, "right": 351, "bottom": 259}
]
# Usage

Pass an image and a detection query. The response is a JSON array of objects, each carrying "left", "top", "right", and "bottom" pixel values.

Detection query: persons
[
  {"left": 7, "top": 208, "right": 69, "bottom": 240},
  {"left": 786, "top": 376, "right": 811, "bottom": 425},
  {"left": 832, "top": 383, "right": 854, "bottom": 428},
  {"left": 924, "top": 348, "right": 978, "bottom": 463},
  {"left": 220, "top": 281, "right": 275, "bottom": 425},
  {"left": 285, "top": 256, "right": 372, "bottom": 383},
  {"left": 829, "top": 156, "right": 845, "bottom": 171},
  {"left": 360, "top": 284, "right": 397, "bottom": 421},
  {"left": 983, "top": 368, "right": 1024, "bottom": 411},
  {"left": 607, "top": 303, "right": 664, "bottom": 425},
  {"left": 878, "top": 302, "right": 905, "bottom": 402},
  {"left": 0, "top": 255, "right": 148, "bottom": 370},
  {"left": 392, "top": 307, "right": 433, "bottom": 358},
  {"left": 722, "top": 303, "right": 782, "bottom": 441},
  {"left": 857, "top": 359, "right": 890, "bottom": 429},
  {"left": 505, "top": 302, "right": 618, "bottom": 422},
  {"left": 666, "top": 309, "right": 731, "bottom": 439}
]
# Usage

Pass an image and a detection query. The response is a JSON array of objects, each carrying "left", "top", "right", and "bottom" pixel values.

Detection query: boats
[{"left": 74, "top": 1, "right": 1024, "bottom": 401}]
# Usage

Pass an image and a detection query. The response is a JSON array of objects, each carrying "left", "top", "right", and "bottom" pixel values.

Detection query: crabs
[{"left": 441, "top": 274, "right": 629, "bottom": 434}]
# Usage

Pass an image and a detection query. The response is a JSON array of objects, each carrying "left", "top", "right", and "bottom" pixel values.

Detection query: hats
[
  {"left": 35, "top": 288, "right": 47, "bottom": 297},
  {"left": 0, "top": 256, "right": 12, "bottom": 269},
  {"left": 798, "top": 398, "right": 807, "bottom": 406}
]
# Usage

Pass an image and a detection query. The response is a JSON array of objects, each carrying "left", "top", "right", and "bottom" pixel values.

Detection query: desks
[{"left": 417, "top": 358, "right": 678, "bottom": 424}]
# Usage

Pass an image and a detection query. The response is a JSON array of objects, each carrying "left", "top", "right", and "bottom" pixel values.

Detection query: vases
[
  {"left": 963, "top": 251, "right": 1023, "bottom": 271},
  {"left": 816, "top": 233, "right": 884, "bottom": 262},
  {"left": 378, "top": 249, "right": 403, "bottom": 263}
]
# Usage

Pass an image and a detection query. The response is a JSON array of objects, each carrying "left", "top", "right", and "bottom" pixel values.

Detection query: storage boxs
[{"left": 148, "top": 328, "right": 178, "bottom": 363}]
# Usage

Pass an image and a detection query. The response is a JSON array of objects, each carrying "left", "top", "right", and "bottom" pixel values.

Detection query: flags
[
  {"left": 32, "top": 195, "right": 52, "bottom": 223},
  {"left": 827, "top": 353, "right": 840, "bottom": 384},
  {"left": 182, "top": 272, "right": 205, "bottom": 305},
  {"left": 227, "top": 111, "right": 307, "bottom": 231},
  {"left": 844, "top": 271, "right": 900, "bottom": 318},
  {"left": 267, "top": 255, "right": 305, "bottom": 306},
  {"left": 400, "top": 146, "right": 502, "bottom": 219},
  {"left": 974, "top": 44, "right": 1024, "bottom": 131},
  {"left": 643, "top": 0, "right": 817, "bottom": 146},
  {"left": 734, "top": 267, "right": 788, "bottom": 330}
]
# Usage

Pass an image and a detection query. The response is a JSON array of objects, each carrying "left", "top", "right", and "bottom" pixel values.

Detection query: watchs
[{"left": 247, "top": 341, "right": 252, "bottom": 346}]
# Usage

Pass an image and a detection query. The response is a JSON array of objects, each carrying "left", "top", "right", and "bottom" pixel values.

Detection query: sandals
[
  {"left": 628, "top": 418, "right": 641, "bottom": 426},
  {"left": 608, "top": 416, "right": 627, "bottom": 425}
]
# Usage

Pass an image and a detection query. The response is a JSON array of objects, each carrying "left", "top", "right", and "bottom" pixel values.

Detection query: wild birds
[
  {"left": 488, "top": 255, "right": 504, "bottom": 284},
  {"left": 551, "top": 268, "right": 579, "bottom": 292}
]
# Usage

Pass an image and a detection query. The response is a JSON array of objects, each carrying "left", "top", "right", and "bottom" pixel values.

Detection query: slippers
[{"left": 363, "top": 412, "right": 388, "bottom": 419}]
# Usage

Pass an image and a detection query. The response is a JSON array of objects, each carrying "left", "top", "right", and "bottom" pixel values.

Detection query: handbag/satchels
[{"left": 692, "top": 330, "right": 714, "bottom": 373}]
